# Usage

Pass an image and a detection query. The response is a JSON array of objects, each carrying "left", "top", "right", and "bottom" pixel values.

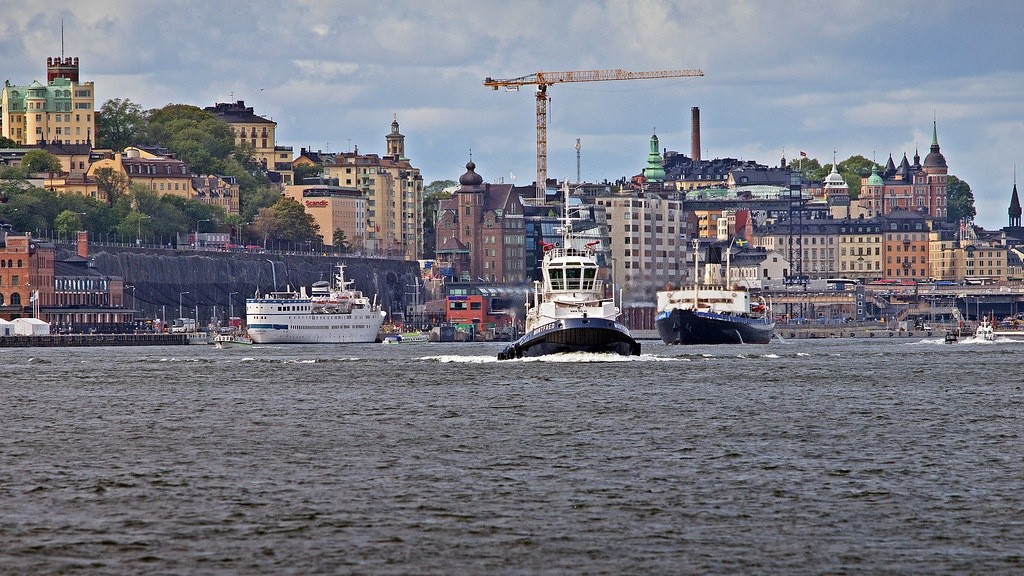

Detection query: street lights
[
  {"left": 139, "top": 215, "right": 151, "bottom": 239},
  {"left": 228, "top": 292, "right": 239, "bottom": 318},
  {"left": 126, "top": 284, "right": 136, "bottom": 310},
  {"left": 180, "top": 291, "right": 189, "bottom": 318},
  {"left": 230, "top": 304, "right": 234, "bottom": 327},
  {"left": 196, "top": 219, "right": 210, "bottom": 249}
]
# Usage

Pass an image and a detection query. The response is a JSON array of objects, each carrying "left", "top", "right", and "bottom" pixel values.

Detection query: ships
[{"left": 246, "top": 262, "right": 387, "bottom": 346}]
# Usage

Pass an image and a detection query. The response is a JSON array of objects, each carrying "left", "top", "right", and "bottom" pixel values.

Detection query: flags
[
  {"left": 30, "top": 291, "right": 38, "bottom": 302},
  {"left": 800, "top": 151, "right": 806, "bottom": 157},
  {"left": 735, "top": 238, "right": 748, "bottom": 247},
  {"left": 510, "top": 172, "right": 517, "bottom": 182}
]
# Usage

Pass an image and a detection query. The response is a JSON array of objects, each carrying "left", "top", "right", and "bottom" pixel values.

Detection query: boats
[
  {"left": 336, "top": 296, "right": 350, "bottom": 304},
  {"left": 498, "top": 178, "right": 643, "bottom": 357},
  {"left": 325, "top": 301, "right": 337, "bottom": 307},
  {"left": 944, "top": 332, "right": 959, "bottom": 346},
  {"left": 653, "top": 237, "right": 776, "bottom": 348},
  {"left": 311, "top": 298, "right": 325, "bottom": 307},
  {"left": 971, "top": 316, "right": 997, "bottom": 341}
]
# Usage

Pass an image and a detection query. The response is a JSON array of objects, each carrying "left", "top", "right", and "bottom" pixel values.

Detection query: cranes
[{"left": 479, "top": 68, "right": 706, "bottom": 195}]
